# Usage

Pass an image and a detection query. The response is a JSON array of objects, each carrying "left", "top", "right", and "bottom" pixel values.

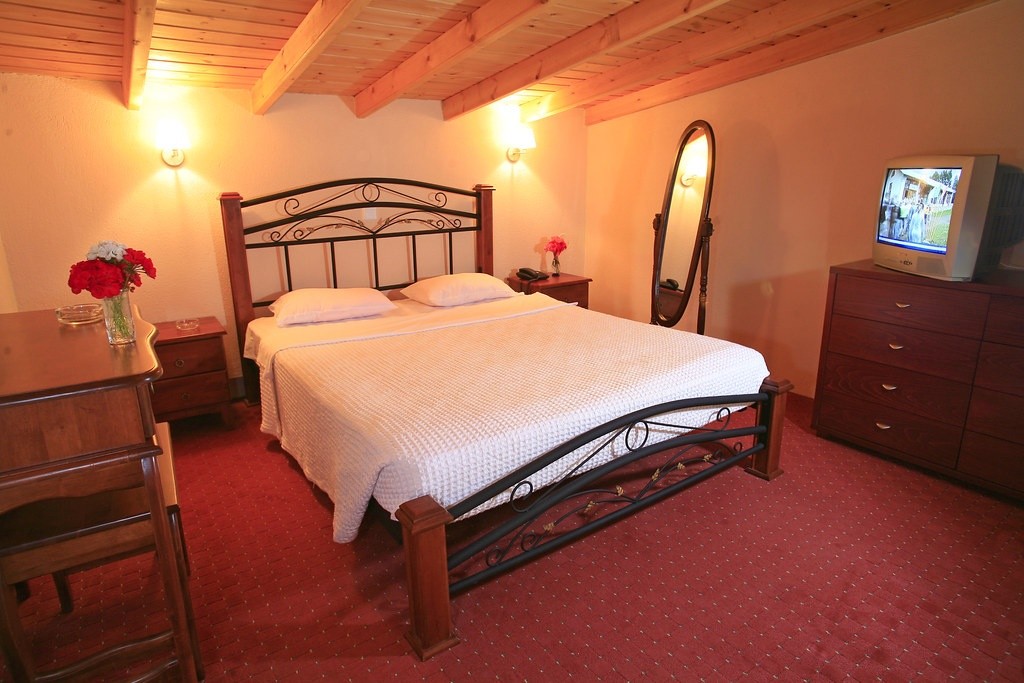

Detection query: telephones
[
  {"left": 516, "top": 268, "right": 549, "bottom": 281},
  {"left": 659, "top": 278, "right": 679, "bottom": 291}
]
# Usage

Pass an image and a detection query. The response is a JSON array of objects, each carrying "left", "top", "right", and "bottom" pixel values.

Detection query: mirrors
[{"left": 650, "top": 118, "right": 715, "bottom": 335}]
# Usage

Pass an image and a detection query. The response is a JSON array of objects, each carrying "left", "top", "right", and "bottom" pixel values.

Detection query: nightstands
[
  {"left": 508, "top": 269, "right": 593, "bottom": 309},
  {"left": 149, "top": 316, "right": 232, "bottom": 433}
]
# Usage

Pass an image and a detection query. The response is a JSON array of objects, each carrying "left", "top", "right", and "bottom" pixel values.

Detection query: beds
[{"left": 219, "top": 177, "right": 791, "bottom": 663}]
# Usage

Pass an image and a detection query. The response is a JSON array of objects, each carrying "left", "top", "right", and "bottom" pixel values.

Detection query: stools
[{"left": 0, "top": 422, "right": 192, "bottom": 683}]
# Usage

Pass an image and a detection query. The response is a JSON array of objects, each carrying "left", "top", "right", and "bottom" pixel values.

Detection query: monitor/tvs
[{"left": 872, "top": 154, "right": 1024, "bottom": 282}]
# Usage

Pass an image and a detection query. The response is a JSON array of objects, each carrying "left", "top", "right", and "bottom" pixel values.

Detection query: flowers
[
  {"left": 70, "top": 242, "right": 157, "bottom": 299},
  {"left": 544, "top": 236, "right": 568, "bottom": 259}
]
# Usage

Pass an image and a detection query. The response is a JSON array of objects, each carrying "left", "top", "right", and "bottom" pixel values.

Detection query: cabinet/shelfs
[{"left": 810, "top": 258, "right": 1024, "bottom": 509}]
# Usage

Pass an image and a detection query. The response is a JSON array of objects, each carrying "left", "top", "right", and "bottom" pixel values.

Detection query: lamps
[
  {"left": 142, "top": 104, "right": 195, "bottom": 167},
  {"left": 499, "top": 124, "right": 536, "bottom": 161}
]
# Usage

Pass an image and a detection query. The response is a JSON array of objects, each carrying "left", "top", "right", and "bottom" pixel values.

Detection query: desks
[{"left": 0, "top": 307, "right": 197, "bottom": 683}]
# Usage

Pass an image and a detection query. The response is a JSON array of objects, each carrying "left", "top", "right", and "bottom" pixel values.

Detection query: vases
[
  {"left": 102, "top": 287, "right": 136, "bottom": 346},
  {"left": 551, "top": 257, "right": 560, "bottom": 276}
]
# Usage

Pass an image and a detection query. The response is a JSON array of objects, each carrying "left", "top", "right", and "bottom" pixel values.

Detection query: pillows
[
  {"left": 401, "top": 272, "right": 524, "bottom": 310},
  {"left": 268, "top": 287, "right": 398, "bottom": 328}
]
